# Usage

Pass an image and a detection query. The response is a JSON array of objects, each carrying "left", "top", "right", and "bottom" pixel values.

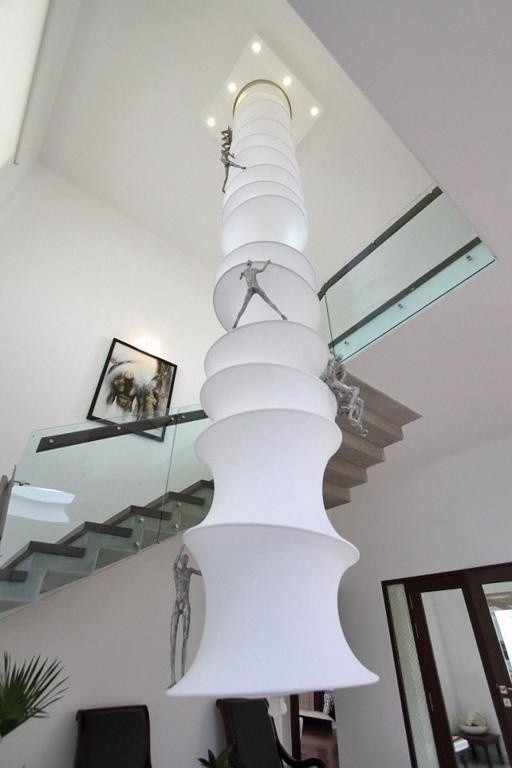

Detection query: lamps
[{"left": 164, "top": 32, "right": 381, "bottom": 700}]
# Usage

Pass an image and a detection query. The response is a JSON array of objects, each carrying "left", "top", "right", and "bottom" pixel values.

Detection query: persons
[
  {"left": 217, "top": 124, "right": 247, "bottom": 192},
  {"left": 164, "top": 541, "right": 202, "bottom": 686},
  {"left": 230, "top": 257, "right": 289, "bottom": 332},
  {"left": 107, "top": 365, "right": 163, "bottom": 423}
]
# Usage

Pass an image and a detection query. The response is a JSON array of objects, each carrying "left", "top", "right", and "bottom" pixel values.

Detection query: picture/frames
[{"left": 84, "top": 335, "right": 180, "bottom": 443}]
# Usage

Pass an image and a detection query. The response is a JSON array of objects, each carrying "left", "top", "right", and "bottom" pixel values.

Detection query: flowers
[{"left": 457, "top": 704, "right": 491, "bottom": 727}]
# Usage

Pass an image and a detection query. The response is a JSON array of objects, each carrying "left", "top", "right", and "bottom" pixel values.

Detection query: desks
[{"left": 455, "top": 732, "right": 504, "bottom": 767}]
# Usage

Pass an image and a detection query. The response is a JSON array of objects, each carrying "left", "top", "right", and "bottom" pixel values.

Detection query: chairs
[
  {"left": 217, "top": 698, "right": 325, "bottom": 767},
  {"left": 69, "top": 702, "right": 154, "bottom": 767}
]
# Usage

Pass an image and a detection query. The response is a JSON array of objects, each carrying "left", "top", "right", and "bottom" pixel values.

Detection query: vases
[{"left": 458, "top": 726, "right": 486, "bottom": 736}]
